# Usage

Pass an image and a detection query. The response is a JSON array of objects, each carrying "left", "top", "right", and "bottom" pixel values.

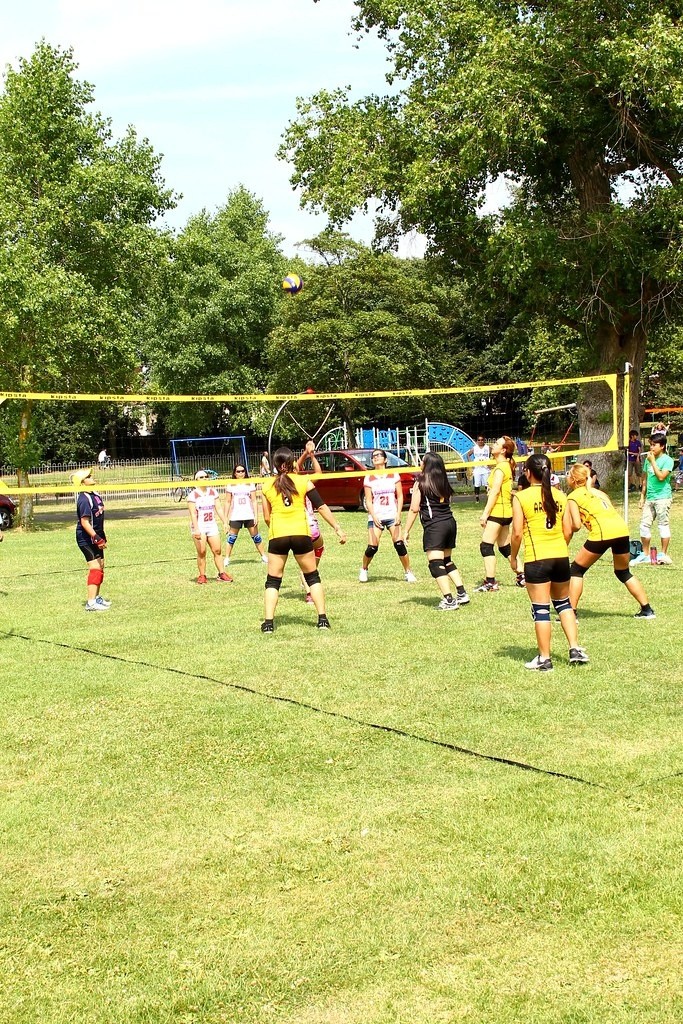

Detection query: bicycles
[{"left": 172, "top": 473, "right": 198, "bottom": 503}]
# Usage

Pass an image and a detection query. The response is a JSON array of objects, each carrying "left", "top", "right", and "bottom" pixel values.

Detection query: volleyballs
[{"left": 283, "top": 273, "right": 304, "bottom": 295}]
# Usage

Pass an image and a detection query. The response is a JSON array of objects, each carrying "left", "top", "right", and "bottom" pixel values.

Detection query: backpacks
[{"left": 629, "top": 540, "right": 644, "bottom": 562}]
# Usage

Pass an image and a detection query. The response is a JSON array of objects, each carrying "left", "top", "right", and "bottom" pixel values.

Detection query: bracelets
[{"left": 335, "top": 524, "right": 341, "bottom": 532}]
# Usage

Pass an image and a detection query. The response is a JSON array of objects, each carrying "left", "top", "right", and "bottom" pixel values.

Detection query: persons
[
  {"left": 97, "top": 447, "right": 111, "bottom": 471},
  {"left": 69, "top": 469, "right": 113, "bottom": 611},
  {"left": 223, "top": 440, "right": 346, "bottom": 634},
  {"left": 404, "top": 451, "right": 471, "bottom": 610},
  {"left": 626, "top": 421, "right": 683, "bottom": 565},
  {"left": 467, "top": 434, "right": 654, "bottom": 672},
  {"left": 359, "top": 449, "right": 418, "bottom": 583},
  {"left": 186, "top": 470, "right": 234, "bottom": 583}
]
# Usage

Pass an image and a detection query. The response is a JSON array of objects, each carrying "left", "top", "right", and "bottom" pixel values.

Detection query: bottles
[{"left": 650, "top": 545, "right": 657, "bottom": 565}]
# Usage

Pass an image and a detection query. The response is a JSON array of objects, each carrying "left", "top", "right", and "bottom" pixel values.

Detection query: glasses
[
  {"left": 371, "top": 453, "right": 383, "bottom": 459},
  {"left": 86, "top": 474, "right": 91, "bottom": 479},
  {"left": 237, "top": 469, "right": 245, "bottom": 473},
  {"left": 199, "top": 476, "right": 209, "bottom": 479},
  {"left": 478, "top": 438, "right": 484, "bottom": 441}
]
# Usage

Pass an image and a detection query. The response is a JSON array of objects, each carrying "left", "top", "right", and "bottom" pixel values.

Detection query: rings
[{"left": 514, "top": 570, "right": 515, "bottom": 572}]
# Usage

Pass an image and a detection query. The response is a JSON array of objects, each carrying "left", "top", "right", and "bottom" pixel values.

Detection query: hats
[{"left": 72, "top": 468, "right": 93, "bottom": 486}]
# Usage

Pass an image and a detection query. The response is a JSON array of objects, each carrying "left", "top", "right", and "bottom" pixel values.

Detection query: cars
[{"left": 297, "top": 448, "right": 424, "bottom": 511}]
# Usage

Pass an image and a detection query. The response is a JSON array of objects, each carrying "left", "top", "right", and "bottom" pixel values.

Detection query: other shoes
[
  {"left": 404, "top": 571, "right": 417, "bottom": 581},
  {"left": 317, "top": 617, "right": 330, "bottom": 630},
  {"left": 476, "top": 499, "right": 479, "bottom": 503},
  {"left": 261, "top": 555, "right": 268, "bottom": 564},
  {"left": 305, "top": 594, "right": 314, "bottom": 605},
  {"left": 657, "top": 552, "right": 665, "bottom": 565},
  {"left": 628, "top": 483, "right": 643, "bottom": 492},
  {"left": 629, "top": 551, "right": 650, "bottom": 566},
  {"left": 261, "top": 622, "right": 274, "bottom": 634},
  {"left": 359, "top": 567, "right": 368, "bottom": 582},
  {"left": 223, "top": 557, "right": 229, "bottom": 566},
  {"left": 671, "top": 488, "right": 678, "bottom": 491}
]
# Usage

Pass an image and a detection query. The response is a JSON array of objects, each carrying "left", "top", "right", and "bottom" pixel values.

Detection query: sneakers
[
  {"left": 433, "top": 591, "right": 471, "bottom": 611},
  {"left": 568, "top": 648, "right": 590, "bottom": 665},
  {"left": 556, "top": 609, "right": 580, "bottom": 624},
  {"left": 514, "top": 574, "right": 526, "bottom": 588},
  {"left": 474, "top": 579, "right": 499, "bottom": 592},
  {"left": 197, "top": 572, "right": 234, "bottom": 584},
  {"left": 634, "top": 606, "right": 657, "bottom": 619},
  {"left": 85, "top": 595, "right": 112, "bottom": 611},
  {"left": 524, "top": 654, "right": 553, "bottom": 670}
]
